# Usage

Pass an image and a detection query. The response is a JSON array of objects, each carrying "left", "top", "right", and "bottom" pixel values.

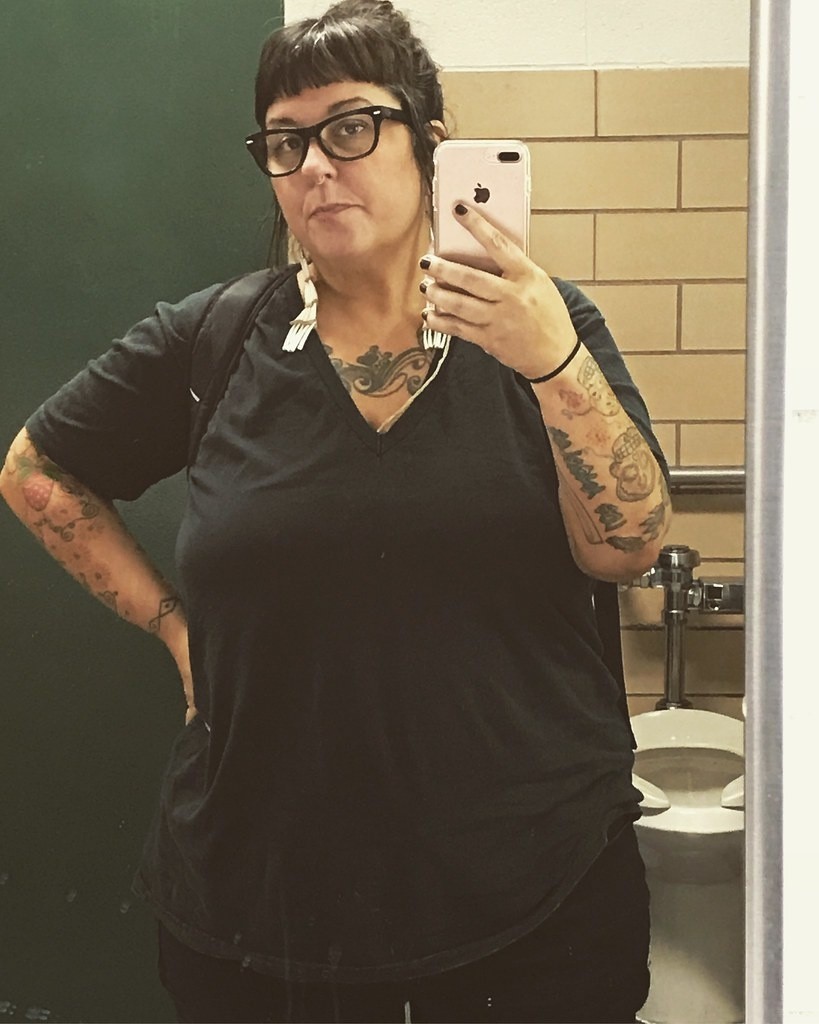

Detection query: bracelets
[{"left": 530, "top": 338, "right": 580, "bottom": 383}]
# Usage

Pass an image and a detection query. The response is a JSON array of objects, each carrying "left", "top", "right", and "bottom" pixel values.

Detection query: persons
[{"left": 0, "top": 0, "right": 674, "bottom": 1024}]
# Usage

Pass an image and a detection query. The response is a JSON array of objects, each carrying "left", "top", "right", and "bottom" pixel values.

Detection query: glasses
[{"left": 244, "top": 106, "right": 411, "bottom": 177}]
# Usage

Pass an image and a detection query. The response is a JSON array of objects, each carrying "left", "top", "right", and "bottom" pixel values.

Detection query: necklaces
[{"left": 375, "top": 335, "right": 452, "bottom": 434}]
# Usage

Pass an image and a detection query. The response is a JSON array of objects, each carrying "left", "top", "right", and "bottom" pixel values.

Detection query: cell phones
[{"left": 432, "top": 140, "right": 532, "bottom": 300}]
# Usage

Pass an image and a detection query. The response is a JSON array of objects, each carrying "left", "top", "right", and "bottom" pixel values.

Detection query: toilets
[{"left": 624, "top": 709, "right": 745, "bottom": 1023}]
